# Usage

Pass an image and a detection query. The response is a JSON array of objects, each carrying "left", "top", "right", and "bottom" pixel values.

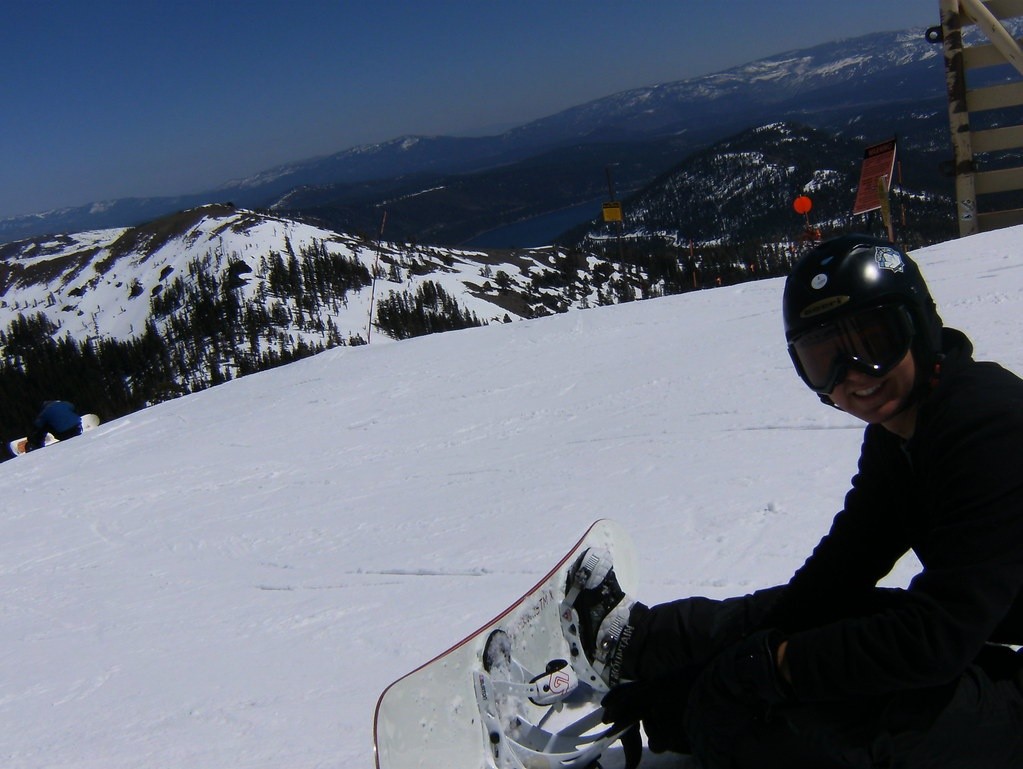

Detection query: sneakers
[{"left": 565, "top": 547, "right": 649, "bottom": 689}]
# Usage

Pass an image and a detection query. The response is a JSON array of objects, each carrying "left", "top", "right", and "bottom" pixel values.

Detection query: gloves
[{"left": 685, "top": 629, "right": 797, "bottom": 757}]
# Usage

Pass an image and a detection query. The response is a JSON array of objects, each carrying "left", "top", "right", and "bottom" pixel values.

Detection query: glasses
[{"left": 787, "top": 305, "right": 912, "bottom": 395}]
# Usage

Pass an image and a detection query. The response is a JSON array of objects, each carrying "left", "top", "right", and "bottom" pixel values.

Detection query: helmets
[{"left": 783, "top": 234, "right": 942, "bottom": 340}]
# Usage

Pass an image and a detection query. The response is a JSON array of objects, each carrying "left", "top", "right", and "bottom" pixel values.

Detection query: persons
[
  {"left": 25, "top": 395, "right": 83, "bottom": 452},
  {"left": 562, "top": 235, "right": 1023, "bottom": 769}
]
